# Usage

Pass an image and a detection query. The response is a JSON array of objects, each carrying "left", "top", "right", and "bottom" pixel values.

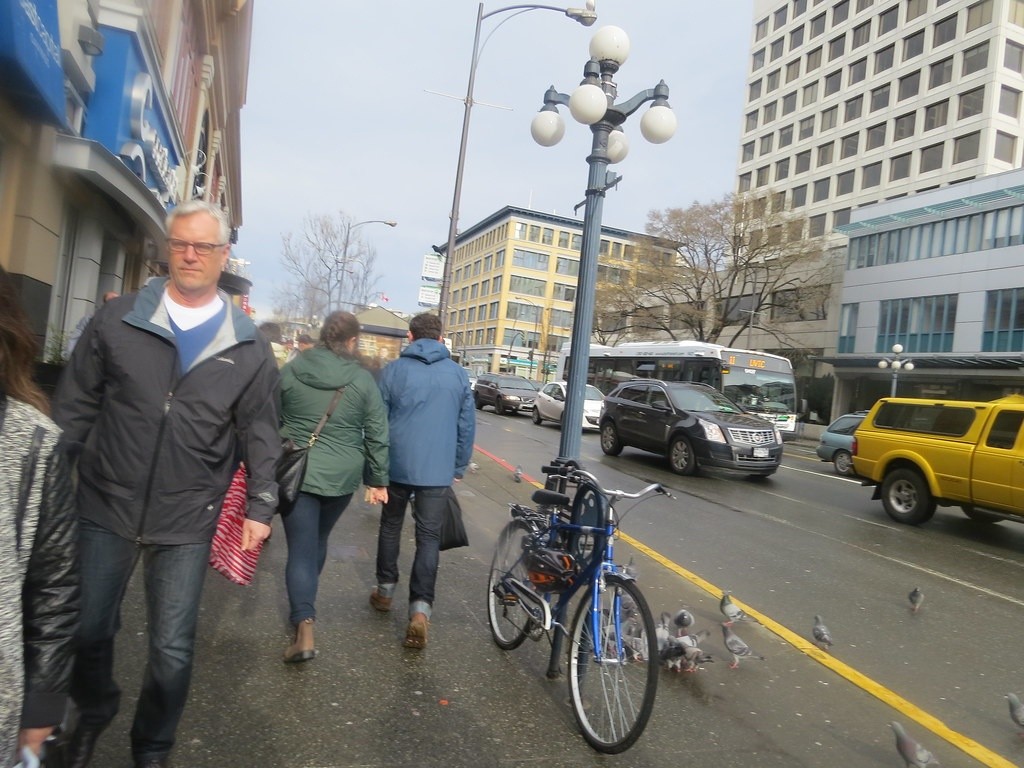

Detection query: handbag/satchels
[
  {"left": 274, "top": 437, "right": 308, "bottom": 503},
  {"left": 410, "top": 489, "right": 469, "bottom": 551}
]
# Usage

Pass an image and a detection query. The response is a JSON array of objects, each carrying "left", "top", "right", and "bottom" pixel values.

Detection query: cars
[
  {"left": 529, "top": 379, "right": 545, "bottom": 390},
  {"left": 532, "top": 381, "right": 606, "bottom": 432}
]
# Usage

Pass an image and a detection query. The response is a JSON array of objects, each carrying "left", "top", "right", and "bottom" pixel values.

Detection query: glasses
[{"left": 165, "top": 239, "right": 226, "bottom": 255}]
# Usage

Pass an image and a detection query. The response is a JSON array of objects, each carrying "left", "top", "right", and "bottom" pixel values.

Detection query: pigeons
[
  {"left": 909, "top": 586, "right": 925, "bottom": 614},
  {"left": 813, "top": 614, "right": 834, "bottom": 649},
  {"left": 597, "top": 590, "right": 767, "bottom": 675},
  {"left": 884, "top": 720, "right": 941, "bottom": 768},
  {"left": 1004, "top": 692, "right": 1024, "bottom": 737}
]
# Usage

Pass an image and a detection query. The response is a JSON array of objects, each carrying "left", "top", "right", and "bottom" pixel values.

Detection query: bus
[{"left": 556, "top": 342, "right": 809, "bottom": 439}]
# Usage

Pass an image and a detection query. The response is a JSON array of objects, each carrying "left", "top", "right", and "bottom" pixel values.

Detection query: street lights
[
  {"left": 530, "top": 25, "right": 679, "bottom": 518},
  {"left": 447, "top": 305, "right": 468, "bottom": 367},
  {"left": 879, "top": 344, "right": 914, "bottom": 400},
  {"left": 440, "top": 1, "right": 598, "bottom": 330},
  {"left": 515, "top": 296, "right": 538, "bottom": 378},
  {"left": 337, "top": 218, "right": 396, "bottom": 312},
  {"left": 722, "top": 251, "right": 756, "bottom": 348}
]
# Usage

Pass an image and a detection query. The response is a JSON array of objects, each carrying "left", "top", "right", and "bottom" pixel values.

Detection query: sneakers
[
  {"left": 403, "top": 613, "right": 430, "bottom": 649},
  {"left": 371, "top": 592, "right": 391, "bottom": 611}
]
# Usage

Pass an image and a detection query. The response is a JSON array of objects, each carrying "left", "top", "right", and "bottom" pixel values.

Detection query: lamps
[{"left": 185, "top": 149, "right": 214, "bottom": 202}]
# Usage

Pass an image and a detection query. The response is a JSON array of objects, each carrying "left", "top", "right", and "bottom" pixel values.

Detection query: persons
[
  {"left": 370, "top": 313, "right": 476, "bottom": 650},
  {"left": 0, "top": 266, "right": 79, "bottom": 768},
  {"left": 279, "top": 311, "right": 391, "bottom": 665},
  {"left": 50, "top": 199, "right": 281, "bottom": 768},
  {"left": 259, "top": 321, "right": 313, "bottom": 368},
  {"left": 750, "top": 386, "right": 769, "bottom": 402}
]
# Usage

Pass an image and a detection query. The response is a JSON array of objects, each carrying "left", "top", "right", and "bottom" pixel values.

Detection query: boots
[{"left": 284, "top": 619, "right": 314, "bottom": 663}]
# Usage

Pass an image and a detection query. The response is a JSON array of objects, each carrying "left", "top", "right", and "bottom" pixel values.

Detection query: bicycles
[{"left": 488, "top": 464, "right": 673, "bottom": 755}]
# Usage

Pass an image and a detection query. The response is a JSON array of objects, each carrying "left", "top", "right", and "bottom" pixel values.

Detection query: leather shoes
[
  {"left": 134, "top": 760, "right": 163, "bottom": 768},
  {"left": 64, "top": 710, "right": 118, "bottom": 768}
]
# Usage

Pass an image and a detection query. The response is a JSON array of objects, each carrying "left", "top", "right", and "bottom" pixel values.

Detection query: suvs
[
  {"left": 475, "top": 373, "right": 539, "bottom": 414},
  {"left": 600, "top": 375, "right": 783, "bottom": 478},
  {"left": 815, "top": 410, "right": 871, "bottom": 476}
]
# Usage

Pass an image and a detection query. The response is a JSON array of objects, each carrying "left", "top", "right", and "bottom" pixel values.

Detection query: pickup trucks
[{"left": 853, "top": 394, "right": 1024, "bottom": 528}]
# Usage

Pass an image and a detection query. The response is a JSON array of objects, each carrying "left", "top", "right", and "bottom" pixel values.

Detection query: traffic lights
[
  {"left": 527, "top": 351, "right": 532, "bottom": 360},
  {"left": 520, "top": 331, "right": 527, "bottom": 342},
  {"left": 463, "top": 351, "right": 466, "bottom": 358}
]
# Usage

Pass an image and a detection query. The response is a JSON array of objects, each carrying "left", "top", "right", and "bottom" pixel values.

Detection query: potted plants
[{"left": 32, "top": 319, "right": 75, "bottom": 395}]
